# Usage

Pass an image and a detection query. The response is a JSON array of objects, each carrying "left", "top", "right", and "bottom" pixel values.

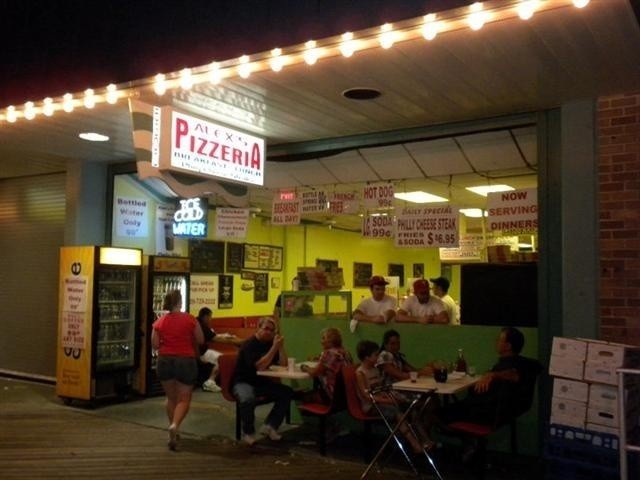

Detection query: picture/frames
[{"left": 185, "top": 237, "right": 284, "bottom": 311}]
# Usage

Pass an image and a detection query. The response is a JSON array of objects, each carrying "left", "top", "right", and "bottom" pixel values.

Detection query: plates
[{"left": 270, "top": 367, "right": 287, "bottom": 372}]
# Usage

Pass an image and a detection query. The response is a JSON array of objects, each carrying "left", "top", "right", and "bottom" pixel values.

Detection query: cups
[
  {"left": 287, "top": 356, "right": 296, "bottom": 374},
  {"left": 410, "top": 372, "right": 417, "bottom": 383},
  {"left": 433, "top": 368, "right": 447, "bottom": 383},
  {"left": 469, "top": 368, "right": 475, "bottom": 377}
]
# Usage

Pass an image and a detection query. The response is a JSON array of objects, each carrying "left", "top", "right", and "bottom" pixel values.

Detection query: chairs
[
  {"left": 202, "top": 313, "right": 294, "bottom": 445},
  {"left": 294, "top": 331, "right": 533, "bottom": 479}
]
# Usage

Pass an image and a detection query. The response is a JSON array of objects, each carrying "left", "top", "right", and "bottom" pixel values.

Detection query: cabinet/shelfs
[{"left": 280, "top": 289, "right": 352, "bottom": 322}]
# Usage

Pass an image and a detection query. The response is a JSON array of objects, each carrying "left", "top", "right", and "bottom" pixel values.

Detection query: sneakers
[
  {"left": 168, "top": 425, "right": 177, "bottom": 450},
  {"left": 259, "top": 424, "right": 281, "bottom": 440},
  {"left": 244, "top": 434, "right": 257, "bottom": 445},
  {"left": 202, "top": 381, "right": 222, "bottom": 392}
]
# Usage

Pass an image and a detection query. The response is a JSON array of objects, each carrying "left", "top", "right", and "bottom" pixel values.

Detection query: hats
[
  {"left": 430, "top": 277, "right": 449, "bottom": 289},
  {"left": 413, "top": 280, "right": 430, "bottom": 293},
  {"left": 369, "top": 276, "right": 387, "bottom": 285}
]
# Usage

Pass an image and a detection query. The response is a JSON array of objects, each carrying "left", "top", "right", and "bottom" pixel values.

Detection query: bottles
[{"left": 456, "top": 349, "right": 467, "bottom": 374}]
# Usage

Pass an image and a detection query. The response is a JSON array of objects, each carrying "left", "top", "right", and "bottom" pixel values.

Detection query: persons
[
  {"left": 374, "top": 329, "right": 444, "bottom": 449},
  {"left": 432, "top": 326, "right": 537, "bottom": 462},
  {"left": 228, "top": 317, "right": 293, "bottom": 448},
  {"left": 151, "top": 290, "right": 205, "bottom": 452},
  {"left": 430, "top": 277, "right": 457, "bottom": 325},
  {"left": 353, "top": 276, "right": 396, "bottom": 326},
  {"left": 354, "top": 340, "right": 433, "bottom": 454},
  {"left": 300, "top": 327, "right": 354, "bottom": 445},
  {"left": 196, "top": 307, "right": 223, "bottom": 392},
  {"left": 394, "top": 279, "right": 450, "bottom": 324}
]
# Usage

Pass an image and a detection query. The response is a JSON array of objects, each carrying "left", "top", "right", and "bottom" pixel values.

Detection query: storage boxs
[{"left": 546, "top": 335, "right": 638, "bottom": 436}]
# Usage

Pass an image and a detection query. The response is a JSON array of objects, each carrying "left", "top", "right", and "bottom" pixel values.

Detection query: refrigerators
[
  {"left": 127, "top": 255, "right": 192, "bottom": 397},
  {"left": 56, "top": 246, "right": 143, "bottom": 409}
]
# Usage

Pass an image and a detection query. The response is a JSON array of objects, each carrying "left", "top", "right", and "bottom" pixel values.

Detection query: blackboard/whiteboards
[{"left": 188, "top": 238, "right": 243, "bottom": 274}]
